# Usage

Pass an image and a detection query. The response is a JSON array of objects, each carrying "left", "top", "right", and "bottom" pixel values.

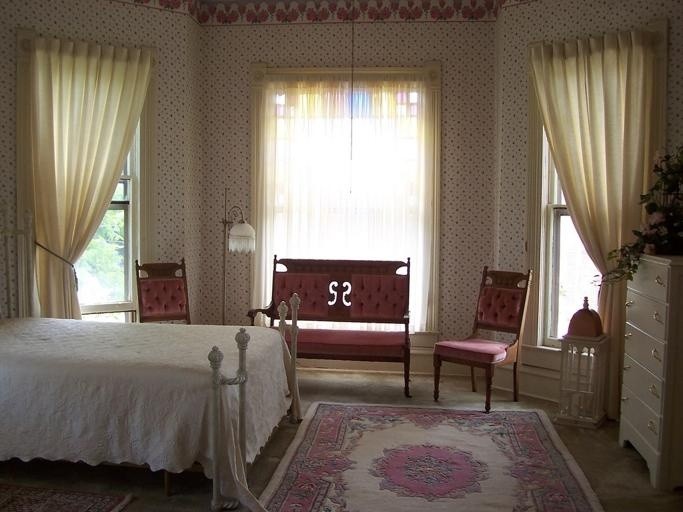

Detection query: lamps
[{"left": 219, "top": 188, "right": 256, "bottom": 325}]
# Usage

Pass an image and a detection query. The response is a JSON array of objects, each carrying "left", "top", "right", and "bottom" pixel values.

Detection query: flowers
[{"left": 605, "top": 144, "right": 683, "bottom": 283}]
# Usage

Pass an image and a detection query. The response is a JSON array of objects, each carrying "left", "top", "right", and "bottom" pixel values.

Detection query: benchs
[{"left": 246, "top": 255, "right": 410, "bottom": 397}]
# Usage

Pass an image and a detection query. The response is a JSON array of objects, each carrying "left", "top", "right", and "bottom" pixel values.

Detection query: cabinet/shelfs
[{"left": 619, "top": 252, "right": 683, "bottom": 495}]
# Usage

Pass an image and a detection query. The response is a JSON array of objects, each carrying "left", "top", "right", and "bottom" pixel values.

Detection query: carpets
[
  {"left": 1, "top": 481, "right": 134, "bottom": 512},
  {"left": 258, "top": 401, "right": 605, "bottom": 512}
]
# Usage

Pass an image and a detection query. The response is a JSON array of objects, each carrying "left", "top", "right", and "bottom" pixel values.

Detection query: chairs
[
  {"left": 135, "top": 257, "right": 192, "bottom": 325},
  {"left": 433, "top": 266, "right": 534, "bottom": 413}
]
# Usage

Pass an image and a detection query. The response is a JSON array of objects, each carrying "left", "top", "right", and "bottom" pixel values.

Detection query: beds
[{"left": 1, "top": 293, "right": 302, "bottom": 512}]
